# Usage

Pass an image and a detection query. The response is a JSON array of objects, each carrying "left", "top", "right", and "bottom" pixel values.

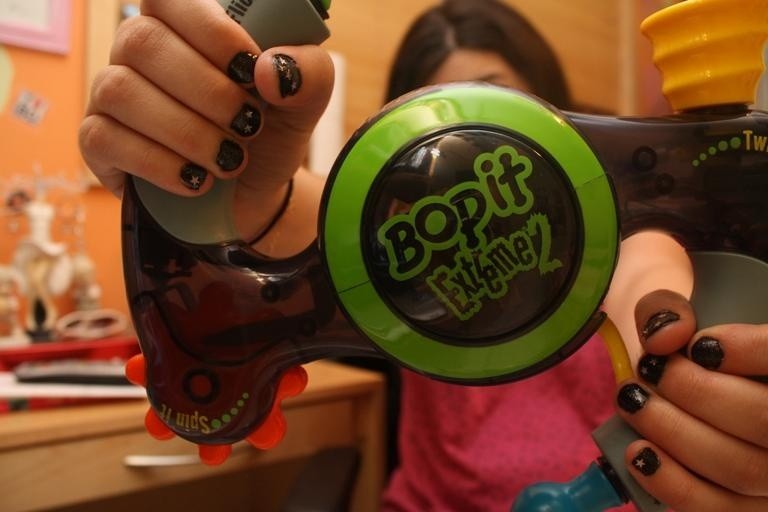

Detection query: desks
[{"left": 0, "top": 356, "right": 389, "bottom": 512}]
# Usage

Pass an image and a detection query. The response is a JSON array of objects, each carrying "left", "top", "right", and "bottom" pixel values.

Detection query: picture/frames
[
  {"left": 0, "top": 0, "right": 75, "bottom": 57},
  {"left": 82, "top": 0, "right": 313, "bottom": 195}
]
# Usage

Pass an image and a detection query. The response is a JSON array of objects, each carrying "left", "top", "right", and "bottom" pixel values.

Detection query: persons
[{"left": 78, "top": 0, "right": 768, "bottom": 512}]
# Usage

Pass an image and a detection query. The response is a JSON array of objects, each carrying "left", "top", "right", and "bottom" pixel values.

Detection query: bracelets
[{"left": 244, "top": 171, "right": 297, "bottom": 251}]
